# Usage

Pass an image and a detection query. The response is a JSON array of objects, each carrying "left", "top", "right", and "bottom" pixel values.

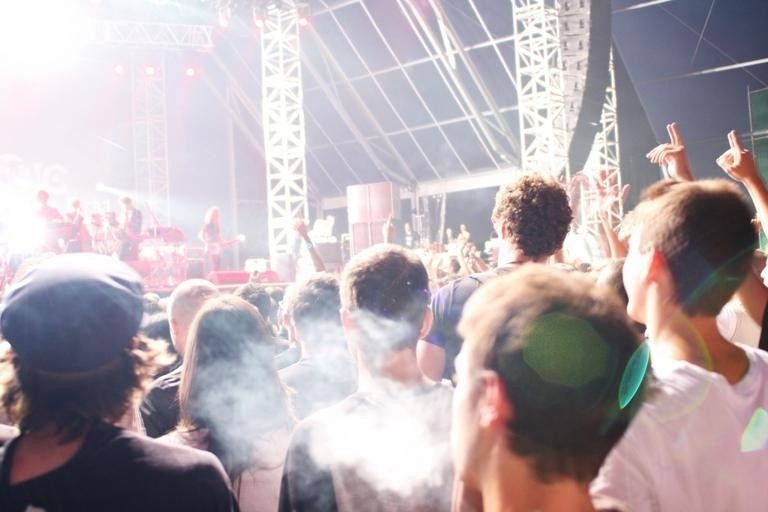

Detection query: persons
[{"left": 0, "top": 119, "right": 767, "bottom": 512}]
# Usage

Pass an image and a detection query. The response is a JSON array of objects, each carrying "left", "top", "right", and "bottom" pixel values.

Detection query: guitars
[{"left": 199, "top": 239, "right": 240, "bottom": 259}]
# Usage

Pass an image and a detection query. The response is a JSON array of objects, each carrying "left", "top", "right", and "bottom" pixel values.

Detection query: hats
[{"left": 0, "top": 249, "right": 146, "bottom": 372}]
